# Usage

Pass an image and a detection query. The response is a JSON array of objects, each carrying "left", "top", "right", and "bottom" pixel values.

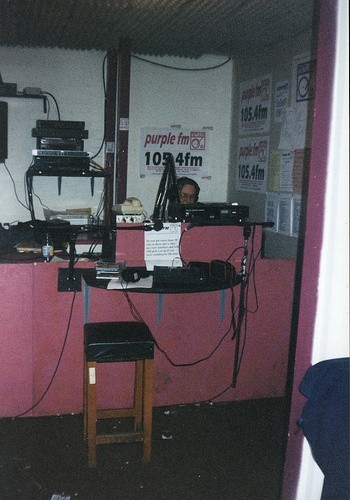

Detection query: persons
[{"left": 149, "top": 177, "right": 200, "bottom": 220}]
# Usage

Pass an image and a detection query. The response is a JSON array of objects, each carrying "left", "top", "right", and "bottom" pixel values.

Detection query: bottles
[{"left": 41, "top": 232, "right": 54, "bottom": 262}]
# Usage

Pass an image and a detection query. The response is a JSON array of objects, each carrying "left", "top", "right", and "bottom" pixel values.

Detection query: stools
[{"left": 82, "top": 321, "right": 156, "bottom": 470}]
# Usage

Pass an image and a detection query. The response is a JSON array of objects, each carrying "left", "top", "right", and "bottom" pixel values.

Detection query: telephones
[{"left": 112, "top": 197, "right": 145, "bottom": 215}]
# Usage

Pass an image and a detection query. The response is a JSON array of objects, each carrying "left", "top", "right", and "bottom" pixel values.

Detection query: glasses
[{"left": 179, "top": 194, "right": 198, "bottom": 201}]
